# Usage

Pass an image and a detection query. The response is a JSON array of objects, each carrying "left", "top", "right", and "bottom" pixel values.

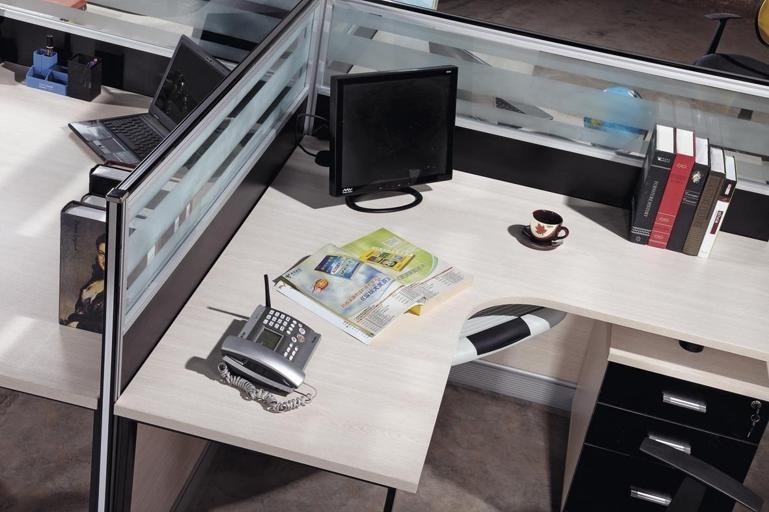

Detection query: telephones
[{"left": 220, "top": 274, "right": 321, "bottom": 393}]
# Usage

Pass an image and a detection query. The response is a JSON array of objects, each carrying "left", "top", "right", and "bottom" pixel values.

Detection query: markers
[{"left": 46, "top": 34, "right": 55, "bottom": 57}]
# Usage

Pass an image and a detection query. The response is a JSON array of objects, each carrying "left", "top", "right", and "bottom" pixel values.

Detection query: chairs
[{"left": 692, "top": 0, "right": 769, "bottom": 83}]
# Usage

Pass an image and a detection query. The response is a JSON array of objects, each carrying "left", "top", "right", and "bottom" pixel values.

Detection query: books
[
  {"left": 648, "top": 128, "right": 693, "bottom": 248},
  {"left": 58, "top": 201, "right": 156, "bottom": 335},
  {"left": 273, "top": 229, "right": 471, "bottom": 344},
  {"left": 103, "top": 160, "right": 194, "bottom": 223},
  {"left": 696, "top": 156, "right": 736, "bottom": 259},
  {"left": 79, "top": 193, "right": 166, "bottom": 267},
  {"left": 681, "top": 146, "right": 726, "bottom": 256},
  {"left": 665, "top": 138, "right": 709, "bottom": 253},
  {"left": 628, "top": 124, "right": 675, "bottom": 245},
  {"left": 88, "top": 163, "right": 181, "bottom": 241}
]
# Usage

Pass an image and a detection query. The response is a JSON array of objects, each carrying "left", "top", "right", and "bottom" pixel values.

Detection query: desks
[
  {"left": 3, "top": 0, "right": 192, "bottom": 51},
  {"left": 109, "top": 129, "right": 769, "bottom": 512},
  {"left": 0, "top": 77, "right": 213, "bottom": 408},
  {"left": 347, "top": 13, "right": 768, "bottom": 186}
]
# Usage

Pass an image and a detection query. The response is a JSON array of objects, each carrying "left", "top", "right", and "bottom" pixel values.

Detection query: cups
[{"left": 529, "top": 208, "right": 570, "bottom": 243}]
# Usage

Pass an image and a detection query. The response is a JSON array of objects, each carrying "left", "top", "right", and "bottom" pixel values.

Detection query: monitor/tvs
[{"left": 329, "top": 64, "right": 458, "bottom": 213}]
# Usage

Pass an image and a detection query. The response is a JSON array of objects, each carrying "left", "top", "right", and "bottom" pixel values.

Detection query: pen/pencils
[{"left": 80, "top": 58, "right": 97, "bottom": 81}]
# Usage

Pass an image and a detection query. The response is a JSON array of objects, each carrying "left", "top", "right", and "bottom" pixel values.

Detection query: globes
[{"left": 583, "top": 86, "right": 649, "bottom": 150}]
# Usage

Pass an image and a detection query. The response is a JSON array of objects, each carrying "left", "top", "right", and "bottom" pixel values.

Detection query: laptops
[
  {"left": 67, "top": 34, "right": 232, "bottom": 167},
  {"left": 429, "top": 41, "right": 553, "bottom": 129}
]
